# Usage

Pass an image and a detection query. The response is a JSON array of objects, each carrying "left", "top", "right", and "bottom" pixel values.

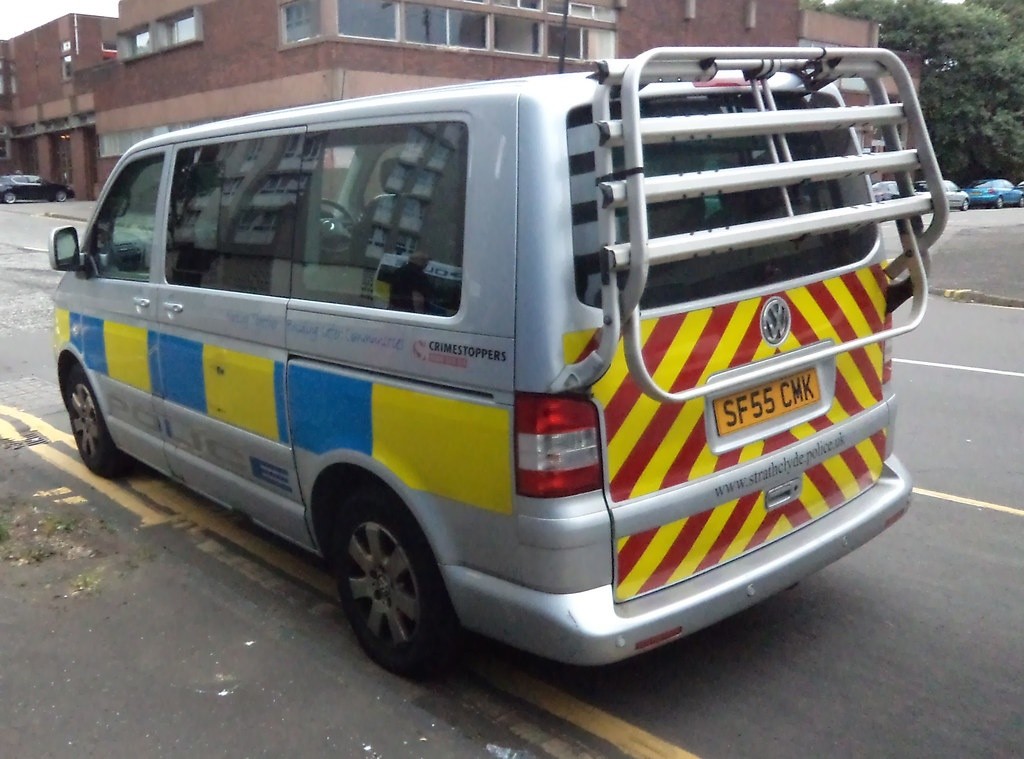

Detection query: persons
[{"left": 389, "top": 250, "right": 435, "bottom": 313}]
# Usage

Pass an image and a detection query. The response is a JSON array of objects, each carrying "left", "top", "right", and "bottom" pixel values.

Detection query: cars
[
  {"left": 1, "top": 174, "right": 75, "bottom": 203},
  {"left": 912, "top": 179, "right": 971, "bottom": 209},
  {"left": 872, "top": 181, "right": 917, "bottom": 201},
  {"left": 963, "top": 178, "right": 1024, "bottom": 208}
]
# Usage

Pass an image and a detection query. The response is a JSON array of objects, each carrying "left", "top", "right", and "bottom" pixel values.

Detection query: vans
[{"left": 48, "top": 43, "right": 951, "bottom": 678}]
